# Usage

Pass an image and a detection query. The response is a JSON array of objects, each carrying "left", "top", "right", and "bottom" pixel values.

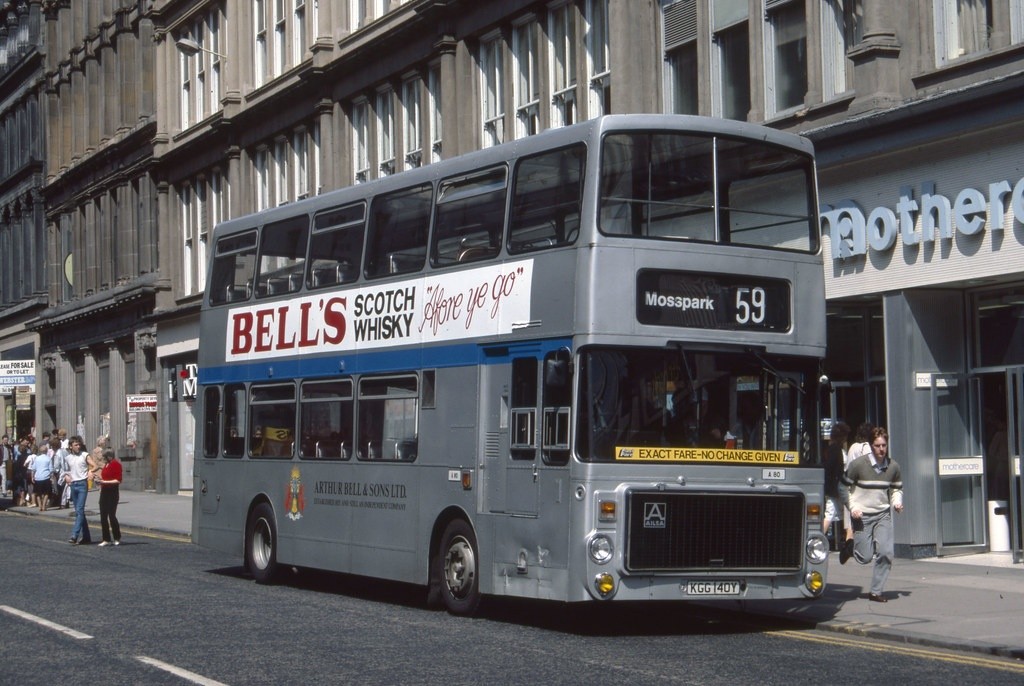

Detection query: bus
[{"left": 188, "top": 110, "right": 832, "bottom": 627}]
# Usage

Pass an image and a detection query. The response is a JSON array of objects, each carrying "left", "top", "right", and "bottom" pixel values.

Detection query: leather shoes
[
  {"left": 869, "top": 594, "right": 887, "bottom": 603},
  {"left": 840, "top": 539, "right": 852, "bottom": 564}
]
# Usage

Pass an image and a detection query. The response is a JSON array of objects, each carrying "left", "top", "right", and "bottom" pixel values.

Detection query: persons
[
  {"left": 0, "top": 429, "right": 87, "bottom": 511},
  {"left": 95, "top": 448, "right": 121, "bottom": 546},
  {"left": 837, "top": 428, "right": 904, "bottom": 603},
  {"left": 822, "top": 424, "right": 847, "bottom": 550},
  {"left": 844, "top": 423, "right": 875, "bottom": 540},
  {"left": 64, "top": 436, "right": 99, "bottom": 545},
  {"left": 92, "top": 436, "right": 107, "bottom": 485}
]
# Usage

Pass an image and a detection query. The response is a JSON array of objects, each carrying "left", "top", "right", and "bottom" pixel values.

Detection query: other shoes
[
  {"left": 115, "top": 538, "right": 121, "bottom": 545},
  {"left": 76, "top": 537, "right": 91, "bottom": 545},
  {"left": 68, "top": 537, "right": 77, "bottom": 543},
  {"left": 99, "top": 540, "right": 112, "bottom": 546}
]
